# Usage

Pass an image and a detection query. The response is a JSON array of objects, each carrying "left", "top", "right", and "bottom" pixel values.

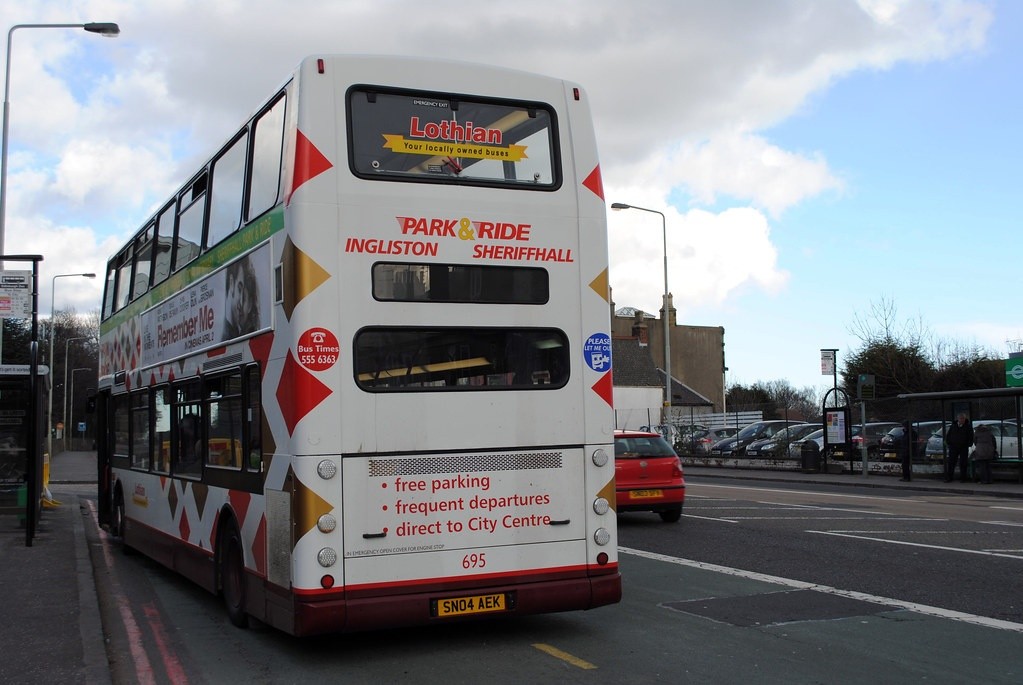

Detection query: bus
[{"left": 96, "top": 52, "right": 622, "bottom": 635}]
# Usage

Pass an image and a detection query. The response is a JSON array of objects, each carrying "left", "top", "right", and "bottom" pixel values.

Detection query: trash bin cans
[{"left": 800, "top": 440, "right": 820, "bottom": 471}]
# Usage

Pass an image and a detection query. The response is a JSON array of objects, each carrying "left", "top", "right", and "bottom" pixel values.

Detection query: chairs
[{"left": 130, "top": 435, "right": 262, "bottom": 479}]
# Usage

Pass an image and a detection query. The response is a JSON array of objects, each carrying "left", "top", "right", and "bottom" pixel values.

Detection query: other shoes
[
  {"left": 898, "top": 478, "right": 911, "bottom": 482},
  {"left": 960, "top": 479, "right": 967, "bottom": 483},
  {"left": 944, "top": 479, "right": 953, "bottom": 483},
  {"left": 978, "top": 480, "right": 993, "bottom": 484}
]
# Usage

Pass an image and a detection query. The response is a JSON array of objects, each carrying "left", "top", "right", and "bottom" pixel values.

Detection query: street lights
[
  {"left": 610, "top": 202, "right": 671, "bottom": 450},
  {"left": 0, "top": 21, "right": 121, "bottom": 270},
  {"left": 63, "top": 337, "right": 94, "bottom": 453},
  {"left": 70, "top": 368, "right": 91, "bottom": 451},
  {"left": 48, "top": 273, "right": 96, "bottom": 461}
]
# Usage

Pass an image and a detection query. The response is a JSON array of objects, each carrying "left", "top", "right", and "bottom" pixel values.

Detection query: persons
[
  {"left": 179, "top": 413, "right": 207, "bottom": 474},
  {"left": 219, "top": 257, "right": 248, "bottom": 343},
  {"left": 944, "top": 412, "right": 974, "bottom": 484},
  {"left": 973, "top": 424, "right": 999, "bottom": 484},
  {"left": 899, "top": 420, "right": 916, "bottom": 482},
  {"left": 236, "top": 270, "right": 259, "bottom": 333}
]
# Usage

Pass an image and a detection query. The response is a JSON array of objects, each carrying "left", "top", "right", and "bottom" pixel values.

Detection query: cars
[
  {"left": 639, "top": 417, "right": 1023, "bottom": 464},
  {"left": 613, "top": 430, "right": 685, "bottom": 523}
]
